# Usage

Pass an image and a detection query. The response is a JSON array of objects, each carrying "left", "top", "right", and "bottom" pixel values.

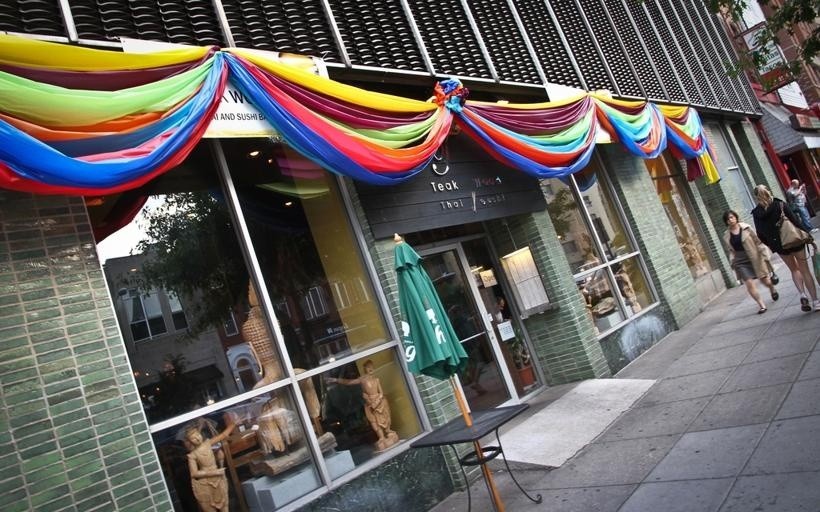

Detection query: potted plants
[{"left": 511, "top": 324, "right": 535, "bottom": 386}]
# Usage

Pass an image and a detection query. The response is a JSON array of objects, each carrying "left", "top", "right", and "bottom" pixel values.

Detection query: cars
[
  {"left": 157, "top": 395, "right": 303, "bottom": 461},
  {"left": 573, "top": 260, "right": 607, "bottom": 289}
]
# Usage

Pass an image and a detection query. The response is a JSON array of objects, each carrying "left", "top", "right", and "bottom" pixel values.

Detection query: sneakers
[
  {"left": 771, "top": 289, "right": 779, "bottom": 301},
  {"left": 813, "top": 300, "right": 820, "bottom": 313},
  {"left": 799, "top": 293, "right": 811, "bottom": 312},
  {"left": 758, "top": 307, "right": 768, "bottom": 314},
  {"left": 809, "top": 228, "right": 819, "bottom": 233}
]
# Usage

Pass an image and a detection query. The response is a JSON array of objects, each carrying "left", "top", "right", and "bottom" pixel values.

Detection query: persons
[
  {"left": 242, "top": 280, "right": 322, "bottom": 455},
  {"left": 169, "top": 399, "right": 218, "bottom": 512},
  {"left": 786, "top": 180, "right": 819, "bottom": 234},
  {"left": 324, "top": 359, "right": 399, "bottom": 449},
  {"left": 491, "top": 297, "right": 507, "bottom": 323},
  {"left": 751, "top": 184, "right": 820, "bottom": 312},
  {"left": 447, "top": 305, "right": 488, "bottom": 395},
  {"left": 723, "top": 209, "right": 781, "bottom": 313},
  {"left": 183, "top": 415, "right": 246, "bottom": 511}
]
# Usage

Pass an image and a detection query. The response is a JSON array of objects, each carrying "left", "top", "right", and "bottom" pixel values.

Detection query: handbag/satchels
[
  {"left": 776, "top": 211, "right": 815, "bottom": 249},
  {"left": 765, "top": 260, "right": 780, "bottom": 285}
]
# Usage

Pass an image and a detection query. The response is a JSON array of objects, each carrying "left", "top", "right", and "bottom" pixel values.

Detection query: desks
[{"left": 410, "top": 403, "right": 542, "bottom": 511}]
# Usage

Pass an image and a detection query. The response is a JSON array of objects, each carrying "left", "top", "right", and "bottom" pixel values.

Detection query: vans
[{"left": 317, "top": 337, "right": 410, "bottom": 435}]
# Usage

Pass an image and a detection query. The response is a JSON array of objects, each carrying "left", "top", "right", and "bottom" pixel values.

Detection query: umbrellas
[{"left": 393, "top": 234, "right": 506, "bottom": 426}]
